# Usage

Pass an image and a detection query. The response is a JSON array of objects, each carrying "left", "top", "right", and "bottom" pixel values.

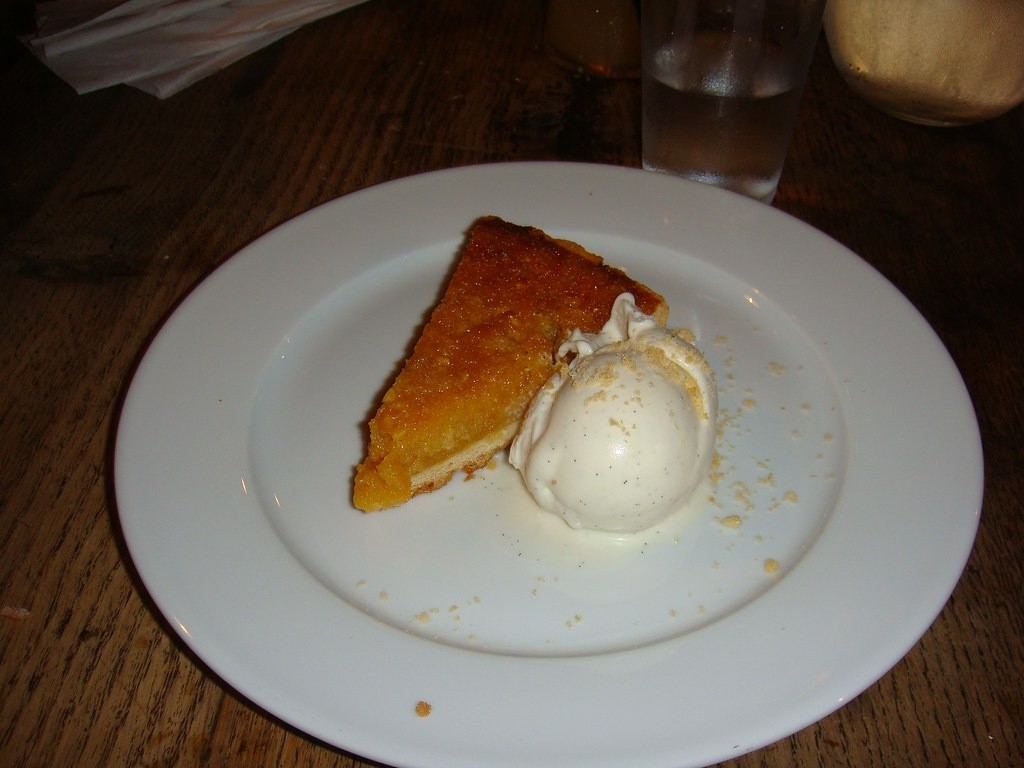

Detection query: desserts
[{"left": 508, "top": 298, "right": 718, "bottom": 534}]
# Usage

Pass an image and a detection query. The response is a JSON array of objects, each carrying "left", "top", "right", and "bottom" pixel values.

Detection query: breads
[{"left": 350, "top": 216, "right": 670, "bottom": 511}]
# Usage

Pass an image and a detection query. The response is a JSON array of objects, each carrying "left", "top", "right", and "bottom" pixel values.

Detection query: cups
[
  {"left": 636, "top": 0, "right": 827, "bottom": 208},
  {"left": 549, "top": 0, "right": 640, "bottom": 78},
  {"left": 823, "top": 0, "right": 1024, "bottom": 127}
]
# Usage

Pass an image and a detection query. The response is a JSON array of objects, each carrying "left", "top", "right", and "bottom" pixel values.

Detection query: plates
[{"left": 114, "top": 162, "right": 985, "bottom": 768}]
